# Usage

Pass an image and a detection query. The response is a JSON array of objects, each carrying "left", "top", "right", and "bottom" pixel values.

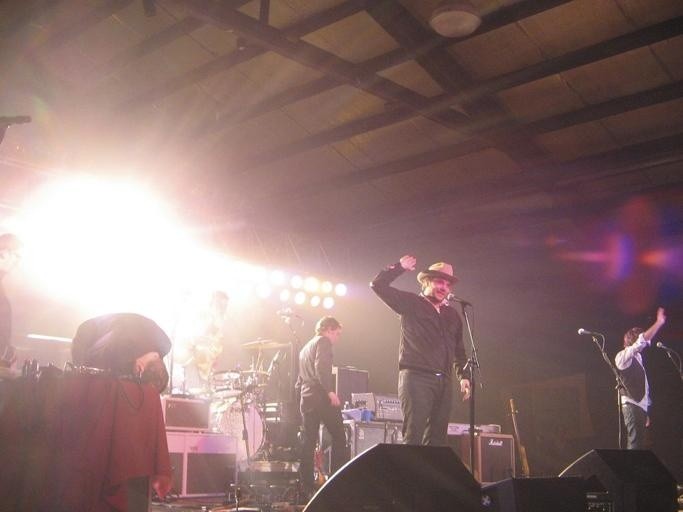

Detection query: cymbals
[{"left": 240, "top": 341, "right": 291, "bottom": 349}]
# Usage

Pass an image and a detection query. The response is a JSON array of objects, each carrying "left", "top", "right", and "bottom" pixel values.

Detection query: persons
[
  {"left": 615, "top": 307, "right": 667, "bottom": 450},
  {"left": 370, "top": 255, "right": 475, "bottom": 446},
  {"left": 71, "top": 313, "right": 172, "bottom": 393},
  {"left": 295, "top": 317, "right": 347, "bottom": 505},
  {"left": 0, "top": 233, "right": 24, "bottom": 382},
  {"left": 163, "top": 291, "right": 230, "bottom": 391}
]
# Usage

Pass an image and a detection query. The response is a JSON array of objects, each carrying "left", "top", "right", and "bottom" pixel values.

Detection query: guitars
[{"left": 507, "top": 399, "right": 530, "bottom": 478}]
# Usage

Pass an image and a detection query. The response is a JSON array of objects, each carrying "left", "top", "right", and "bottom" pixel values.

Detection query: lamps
[{"left": 430, "top": 3, "right": 479, "bottom": 40}]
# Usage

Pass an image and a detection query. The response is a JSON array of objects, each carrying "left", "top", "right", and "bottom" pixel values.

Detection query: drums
[{"left": 207, "top": 370, "right": 272, "bottom": 460}]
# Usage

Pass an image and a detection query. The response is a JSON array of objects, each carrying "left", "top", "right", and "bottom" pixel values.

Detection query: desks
[{"left": 0, "top": 366, "right": 171, "bottom": 512}]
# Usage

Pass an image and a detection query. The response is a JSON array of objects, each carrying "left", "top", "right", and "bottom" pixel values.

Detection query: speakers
[
  {"left": 558, "top": 449, "right": 679, "bottom": 512},
  {"left": 150, "top": 396, "right": 238, "bottom": 498},
  {"left": 343, "top": 420, "right": 404, "bottom": 461},
  {"left": 460, "top": 432, "right": 515, "bottom": 488},
  {"left": 480, "top": 476, "right": 587, "bottom": 512},
  {"left": 302, "top": 443, "right": 483, "bottom": 512}
]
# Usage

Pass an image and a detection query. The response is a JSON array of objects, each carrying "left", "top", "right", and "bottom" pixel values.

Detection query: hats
[{"left": 417, "top": 262, "right": 457, "bottom": 284}]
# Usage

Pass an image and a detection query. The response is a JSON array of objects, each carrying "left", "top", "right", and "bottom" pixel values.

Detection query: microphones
[
  {"left": 447, "top": 294, "right": 469, "bottom": 305},
  {"left": 656, "top": 341, "right": 674, "bottom": 353},
  {"left": 277, "top": 310, "right": 303, "bottom": 320},
  {"left": 577, "top": 328, "right": 600, "bottom": 336},
  {"left": 236, "top": 384, "right": 253, "bottom": 400}
]
